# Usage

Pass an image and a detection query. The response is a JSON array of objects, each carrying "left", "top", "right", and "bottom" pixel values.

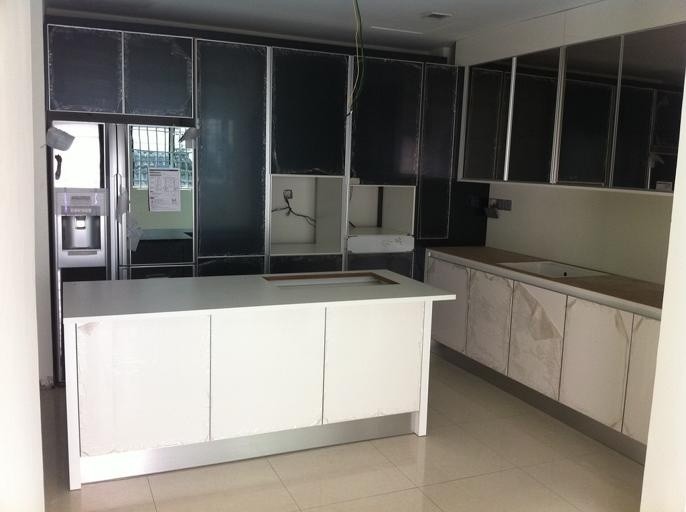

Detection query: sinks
[{"left": 494, "top": 259, "right": 610, "bottom": 281}]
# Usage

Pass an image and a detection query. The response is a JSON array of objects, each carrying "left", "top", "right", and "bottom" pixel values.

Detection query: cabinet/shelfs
[{"left": 55, "top": 265, "right": 466, "bottom": 494}]
